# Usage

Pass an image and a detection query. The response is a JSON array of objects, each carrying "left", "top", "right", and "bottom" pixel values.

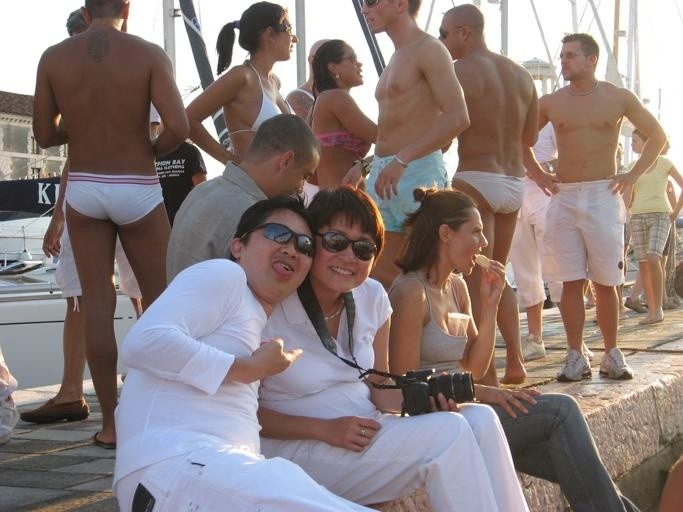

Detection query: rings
[{"left": 362, "top": 427, "right": 365, "bottom": 436}]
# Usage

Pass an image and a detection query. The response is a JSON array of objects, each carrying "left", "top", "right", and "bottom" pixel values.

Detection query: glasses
[
  {"left": 440, "top": 26, "right": 465, "bottom": 38},
  {"left": 358, "top": 0, "right": 379, "bottom": 7},
  {"left": 332, "top": 54, "right": 358, "bottom": 64},
  {"left": 315, "top": 230, "right": 377, "bottom": 261},
  {"left": 241, "top": 223, "right": 315, "bottom": 257},
  {"left": 263, "top": 23, "right": 292, "bottom": 33}
]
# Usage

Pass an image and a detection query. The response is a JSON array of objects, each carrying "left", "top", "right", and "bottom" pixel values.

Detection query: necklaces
[{"left": 325, "top": 298, "right": 345, "bottom": 321}]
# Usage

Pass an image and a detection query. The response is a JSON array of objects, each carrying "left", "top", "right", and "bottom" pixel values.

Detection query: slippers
[
  {"left": 94, "top": 432, "right": 117, "bottom": 448},
  {"left": 585, "top": 303, "right": 596, "bottom": 309}
]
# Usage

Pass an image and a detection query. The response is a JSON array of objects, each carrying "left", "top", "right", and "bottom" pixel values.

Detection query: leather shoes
[{"left": 21, "top": 399, "right": 90, "bottom": 422}]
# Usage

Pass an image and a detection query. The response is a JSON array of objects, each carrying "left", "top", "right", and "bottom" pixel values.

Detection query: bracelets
[
  {"left": 473, "top": 384, "right": 484, "bottom": 402},
  {"left": 392, "top": 153, "right": 408, "bottom": 168}
]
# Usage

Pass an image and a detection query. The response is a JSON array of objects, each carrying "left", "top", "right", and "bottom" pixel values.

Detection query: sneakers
[
  {"left": 557, "top": 350, "right": 591, "bottom": 381},
  {"left": 624, "top": 301, "right": 647, "bottom": 313},
  {"left": 599, "top": 347, "right": 633, "bottom": 379},
  {"left": 522, "top": 340, "right": 546, "bottom": 360},
  {"left": 582, "top": 342, "right": 593, "bottom": 360},
  {"left": 0, "top": 398, "right": 20, "bottom": 444}
]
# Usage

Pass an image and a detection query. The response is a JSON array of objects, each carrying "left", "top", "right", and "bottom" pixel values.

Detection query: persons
[
  {"left": 436, "top": 3, "right": 540, "bottom": 386},
  {"left": 256, "top": 186, "right": 531, "bottom": 512},
  {"left": 184, "top": 1, "right": 300, "bottom": 166},
  {"left": 154, "top": 142, "right": 208, "bottom": 231},
  {"left": 582, "top": 280, "right": 595, "bottom": 309},
  {"left": 167, "top": 113, "right": 322, "bottom": 286},
  {"left": 509, "top": 122, "right": 595, "bottom": 361},
  {"left": 521, "top": 33, "right": 666, "bottom": 382},
  {"left": 21, "top": 5, "right": 132, "bottom": 424},
  {"left": 307, "top": 39, "right": 378, "bottom": 195},
  {"left": 150, "top": 106, "right": 162, "bottom": 142},
  {"left": 657, "top": 455, "right": 683, "bottom": 512},
  {"left": 113, "top": 196, "right": 378, "bottom": 512},
  {"left": 286, "top": 40, "right": 327, "bottom": 125},
  {"left": 389, "top": 187, "right": 640, "bottom": 512},
  {"left": 625, "top": 134, "right": 676, "bottom": 314},
  {"left": 342, "top": 0, "right": 473, "bottom": 290},
  {"left": 627, "top": 128, "right": 683, "bottom": 325},
  {"left": 32, "top": 0, "right": 184, "bottom": 449}
]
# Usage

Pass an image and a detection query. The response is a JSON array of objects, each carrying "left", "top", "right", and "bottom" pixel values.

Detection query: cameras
[{"left": 402, "top": 368, "right": 476, "bottom": 415}]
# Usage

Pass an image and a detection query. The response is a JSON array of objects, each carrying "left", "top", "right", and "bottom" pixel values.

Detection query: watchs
[{"left": 352, "top": 158, "right": 372, "bottom": 177}]
